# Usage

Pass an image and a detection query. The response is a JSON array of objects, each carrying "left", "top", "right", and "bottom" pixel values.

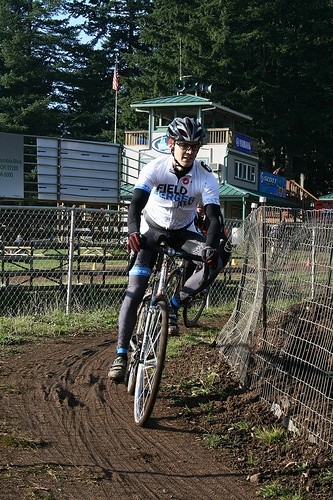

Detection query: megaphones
[
  {"left": 174, "top": 80, "right": 185, "bottom": 92},
  {"left": 184, "top": 79, "right": 195, "bottom": 91},
  {"left": 195, "top": 81, "right": 212, "bottom": 93}
]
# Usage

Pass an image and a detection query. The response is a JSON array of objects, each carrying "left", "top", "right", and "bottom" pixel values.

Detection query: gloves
[
  {"left": 126, "top": 233, "right": 145, "bottom": 252},
  {"left": 201, "top": 246, "right": 218, "bottom": 268}
]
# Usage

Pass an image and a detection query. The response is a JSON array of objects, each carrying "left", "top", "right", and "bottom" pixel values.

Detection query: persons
[
  {"left": 174, "top": 204, "right": 227, "bottom": 299},
  {"left": 106, "top": 116, "right": 229, "bottom": 382}
]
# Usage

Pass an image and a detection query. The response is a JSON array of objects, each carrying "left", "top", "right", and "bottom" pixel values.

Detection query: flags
[{"left": 112, "top": 63, "right": 120, "bottom": 91}]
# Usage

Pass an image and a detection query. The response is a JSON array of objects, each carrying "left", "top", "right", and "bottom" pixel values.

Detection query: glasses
[{"left": 176, "top": 142, "right": 202, "bottom": 150}]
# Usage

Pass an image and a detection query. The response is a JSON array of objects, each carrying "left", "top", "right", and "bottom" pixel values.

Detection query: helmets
[{"left": 166, "top": 118, "right": 205, "bottom": 141}]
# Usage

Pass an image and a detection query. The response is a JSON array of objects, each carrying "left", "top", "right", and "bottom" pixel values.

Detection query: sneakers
[
  {"left": 107, "top": 357, "right": 127, "bottom": 379},
  {"left": 164, "top": 305, "right": 179, "bottom": 336}
]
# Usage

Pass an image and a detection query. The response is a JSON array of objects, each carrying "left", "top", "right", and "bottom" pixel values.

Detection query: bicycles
[{"left": 123, "top": 241, "right": 214, "bottom": 427}]
